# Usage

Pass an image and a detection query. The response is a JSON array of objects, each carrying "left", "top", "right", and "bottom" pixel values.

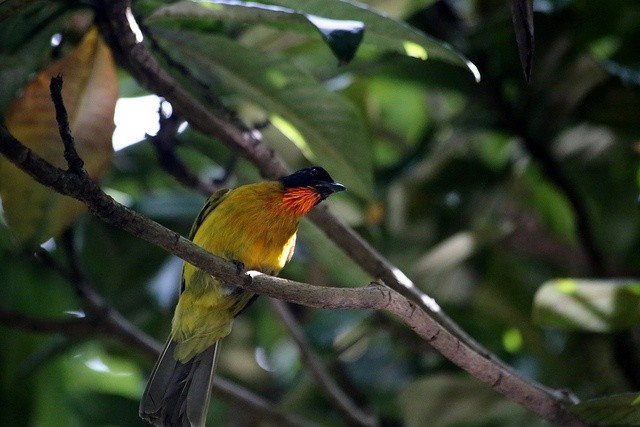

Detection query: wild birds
[{"left": 139, "top": 167, "right": 346, "bottom": 426}]
